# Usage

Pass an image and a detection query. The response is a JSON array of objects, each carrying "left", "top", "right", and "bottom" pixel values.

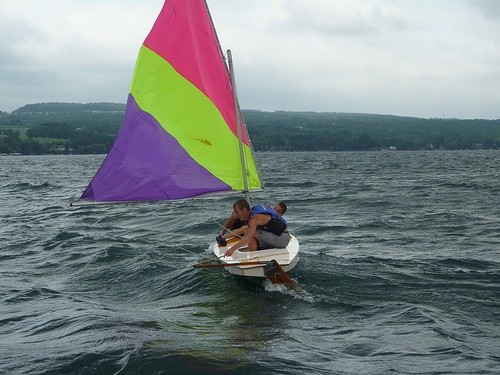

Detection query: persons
[
  {"left": 223, "top": 199, "right": 290, "bottom": 258},
  {"left": 216, "top": 202, "right": 287, "bottom": 246}
]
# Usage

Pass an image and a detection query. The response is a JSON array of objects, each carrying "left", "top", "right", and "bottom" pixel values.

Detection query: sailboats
[{"left": 77, "top": 0, "right": 300, "bottom": 290}]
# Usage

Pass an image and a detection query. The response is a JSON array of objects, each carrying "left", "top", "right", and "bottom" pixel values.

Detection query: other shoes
[{"left": 216, "top": 235, "right": 225, "bottom": 244}]
